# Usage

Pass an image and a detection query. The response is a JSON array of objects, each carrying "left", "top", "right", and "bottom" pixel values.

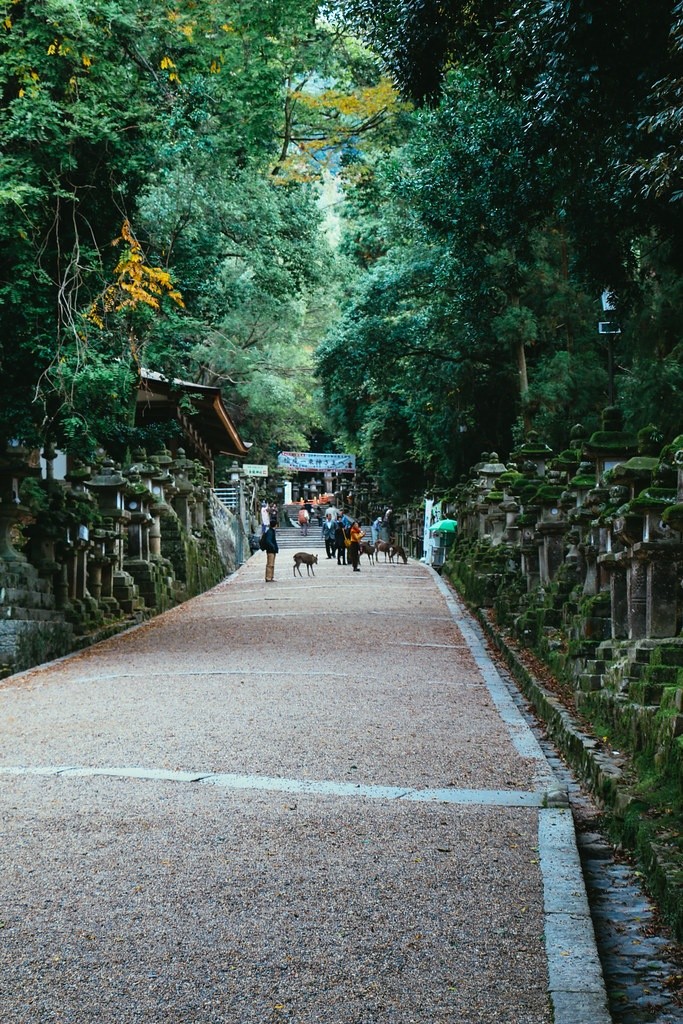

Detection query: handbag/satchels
[{"left": 344, "top": 538, "right": 351, "bottom": 547}]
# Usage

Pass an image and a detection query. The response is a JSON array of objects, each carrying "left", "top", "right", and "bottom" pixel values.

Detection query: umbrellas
[{"left": 428, "top": 518, "right": 457, "bottom": 563}]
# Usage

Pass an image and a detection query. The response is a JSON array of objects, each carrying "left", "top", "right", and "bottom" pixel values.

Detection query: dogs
[
  {"left": 358, "top": 540, "right": 408, "bottom": 566},
  {"left": 292, "top": 552, "right": 319, "bottom": 578}
]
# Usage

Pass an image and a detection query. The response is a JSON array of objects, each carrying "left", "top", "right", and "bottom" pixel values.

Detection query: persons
[
  {"left": 298, "top": 500, "right": 393, "bottom": 572},
  {"left": 260, "top": 502, "right": 278, "bottom": 534},
  {"left": 264, "top": 520, "right": 278, "bottom": 582}
]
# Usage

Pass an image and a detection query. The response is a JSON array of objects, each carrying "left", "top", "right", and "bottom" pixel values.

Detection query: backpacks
[{"left": 259, "top": 531, "right": 273, "bottom": 550}]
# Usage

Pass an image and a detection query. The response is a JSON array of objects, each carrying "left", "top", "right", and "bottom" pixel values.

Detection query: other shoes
[
  {"left": 353, "top": 568, "right": 359, "bottom": 571},
  {"left": 266, "top": 579, "right": 276, "bottom": 582}
]
